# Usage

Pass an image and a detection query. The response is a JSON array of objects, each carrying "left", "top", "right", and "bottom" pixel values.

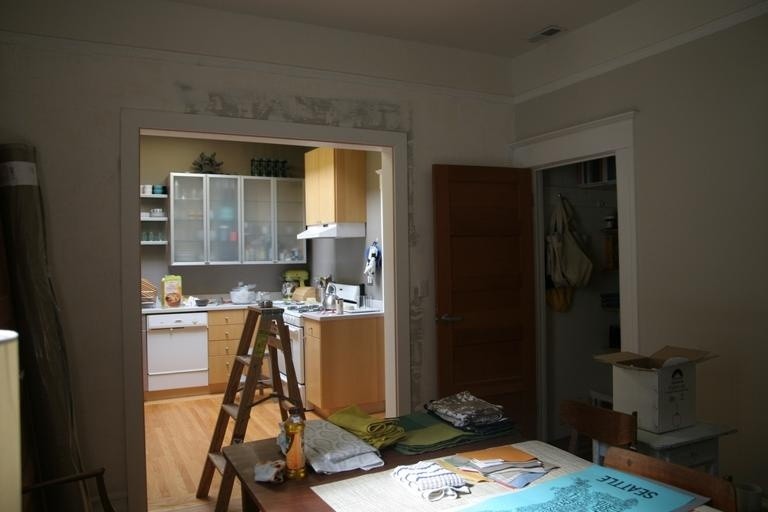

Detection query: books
[{"left": 437, "top": 444, "right": 560, "bottom": 492}]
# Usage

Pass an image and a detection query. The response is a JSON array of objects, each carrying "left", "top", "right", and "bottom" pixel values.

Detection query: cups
[
  {"left": 141, "top": 232, "right": 163, "bottom": 242},
  {"left": 356, "top": 295, "right": 373, "bottom": 310}
]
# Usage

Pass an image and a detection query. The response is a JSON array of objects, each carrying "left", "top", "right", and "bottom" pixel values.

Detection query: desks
[
  {"left": 634, "top": 418, "right": 743, "bottom": 483},
  {"left": 220, "top": 412, "right": 732, "bottom": 512}
]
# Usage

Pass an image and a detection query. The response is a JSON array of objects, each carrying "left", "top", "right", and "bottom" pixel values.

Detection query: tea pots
[{"left": 321, "top": 284, "right": 339, "bottom": 311}]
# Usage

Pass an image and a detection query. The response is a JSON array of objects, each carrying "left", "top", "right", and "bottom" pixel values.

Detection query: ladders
[{"left": 195, "top": 304, "right": 308, "bottom": 512}]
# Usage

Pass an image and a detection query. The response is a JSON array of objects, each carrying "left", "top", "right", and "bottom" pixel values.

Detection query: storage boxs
[{"left": 591, "top": 342, "right": 722, "bottom": 435}]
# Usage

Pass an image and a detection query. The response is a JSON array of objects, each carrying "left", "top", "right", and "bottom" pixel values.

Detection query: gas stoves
[{"left": 274, "top": 300, "right": 354, "bottom": 329}]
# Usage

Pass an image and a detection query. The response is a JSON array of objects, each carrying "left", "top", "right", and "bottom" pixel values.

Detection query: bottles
[
  {"left": 335, "top": 298, "right": 343, "bottom": 315},
  {"left": 283, "top": 408, "right": 306, "bottom": 481}
]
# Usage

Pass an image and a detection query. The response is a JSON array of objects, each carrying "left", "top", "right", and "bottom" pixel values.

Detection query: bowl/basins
[
  {"left": 229, "top": 291, "right": 254, "bottom": 304},
  {"left": 195, "top": 300, "right": 210, "bottom": 306}
]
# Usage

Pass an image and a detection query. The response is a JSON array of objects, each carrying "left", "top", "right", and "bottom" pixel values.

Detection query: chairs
[
  {"left": 600, "top": 445, "right": 737, "bottom": 512},
  {"left": 557, "top": 399, "right": 640, "bottom": 465}
]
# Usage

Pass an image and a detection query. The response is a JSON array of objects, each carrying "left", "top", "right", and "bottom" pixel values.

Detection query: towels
[
  {"left": 277, "top": 391, "right": 515, "bottom": 502},
  {"left": 363, "top": 246, "right": 377, "bottom": 286}
]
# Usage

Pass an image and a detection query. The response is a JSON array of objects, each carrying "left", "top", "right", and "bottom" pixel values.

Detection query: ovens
[{"left": 273, "top": 322, "right": 314, "bottom": 412}]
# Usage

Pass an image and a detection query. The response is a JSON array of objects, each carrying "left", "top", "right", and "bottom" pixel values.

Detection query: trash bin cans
[{"left": 735, "top": 482, "right": 764, "bottom": 512}]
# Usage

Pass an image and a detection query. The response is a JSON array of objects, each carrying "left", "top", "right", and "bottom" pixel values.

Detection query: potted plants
[{"left": 317, "top": 274, "right": 332, "bottom": 306}]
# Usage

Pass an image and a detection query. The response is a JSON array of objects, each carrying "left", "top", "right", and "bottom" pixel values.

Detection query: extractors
[{"left": 296, "top": 222, "right": 366, "bottom": 240}]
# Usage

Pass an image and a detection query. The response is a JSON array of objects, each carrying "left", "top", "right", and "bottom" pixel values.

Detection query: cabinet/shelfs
[
  {"left": 303, "top": 318, "right": 386, "bottom": 414},
  {"left": 167, "top": 172, "right": 243, "bottom": 268},
  {"left": 139, "top": 193, "right": 167, "bottom": 248},
  {"left": 303, "top": 142, "right": 370, "bottom": 228},
  {"left": 208, "top": 308, "right": 244, "bottom": 389},
  {"left": 241, "top": 175, "right": 308, "bottom": 267}
]
